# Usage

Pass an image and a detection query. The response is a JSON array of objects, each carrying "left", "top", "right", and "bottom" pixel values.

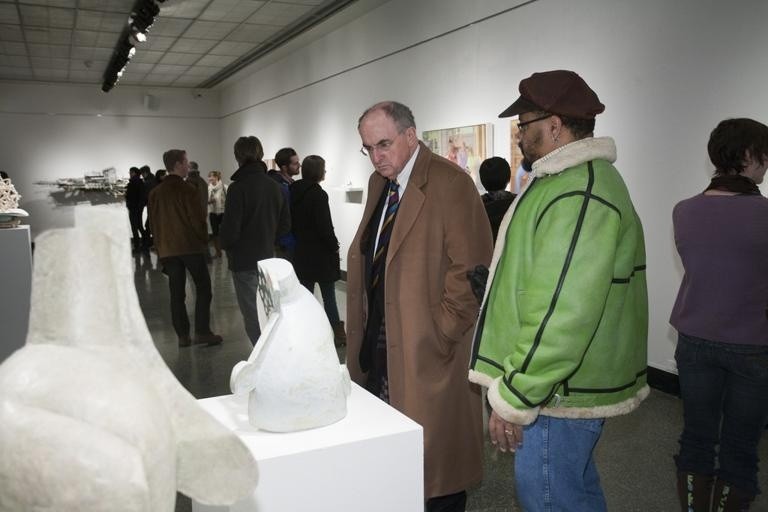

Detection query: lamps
[{"left": 101, "top": 0, "right": 169, "bottom": 93}]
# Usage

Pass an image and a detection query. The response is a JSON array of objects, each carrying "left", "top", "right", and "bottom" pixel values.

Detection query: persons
[
  {"left": 467, "top": 69, "right": 651, "bottom": 511},
  {"left": 669, "top": 117, "right": 768, "bottom": 510},
  {"left": 126, "top": 165, "right": 166, "bottom": 256},
  {"left": 149, "top": 136, "right": 346, "bottom": 349},
  {"left": 479, "top": 156, "right": 517, "bottom": 238},
  {"left": 344, "top": 102, "right": 493, "bottom": 511}
]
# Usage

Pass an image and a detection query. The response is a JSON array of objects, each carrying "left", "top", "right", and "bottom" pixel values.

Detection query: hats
[{"left": 498, "top": 70, "right": 605, "bottom": 118}]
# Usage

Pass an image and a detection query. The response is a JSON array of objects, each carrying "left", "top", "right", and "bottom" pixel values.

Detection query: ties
[{"left": 372, "top": 179, "right": 400, "bottom": 288}]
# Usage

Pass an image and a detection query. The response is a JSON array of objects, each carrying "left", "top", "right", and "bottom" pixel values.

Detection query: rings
[{"left": 504, "top": 430, "right": 513, "bottom": 435}]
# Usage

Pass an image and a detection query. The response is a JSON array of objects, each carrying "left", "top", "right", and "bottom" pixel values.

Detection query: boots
[{"left": 678, "top": 480, "right": 752, "bottom": 512}]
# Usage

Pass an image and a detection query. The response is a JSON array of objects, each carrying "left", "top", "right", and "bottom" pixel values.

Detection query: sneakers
[
  {"left": 193, "top": 334, "right": 223, "bottom": 344},
  {"left": 179, "top": 333, "right": 191, "bottom": 345}
]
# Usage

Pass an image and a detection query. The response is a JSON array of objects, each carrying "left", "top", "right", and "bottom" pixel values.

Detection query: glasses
[
  {"left": 516, "top": 113, "right": 555, "bottom": 133},
  {"left": 360, "top": 128, "right": 402, "bottom": 156}
]
